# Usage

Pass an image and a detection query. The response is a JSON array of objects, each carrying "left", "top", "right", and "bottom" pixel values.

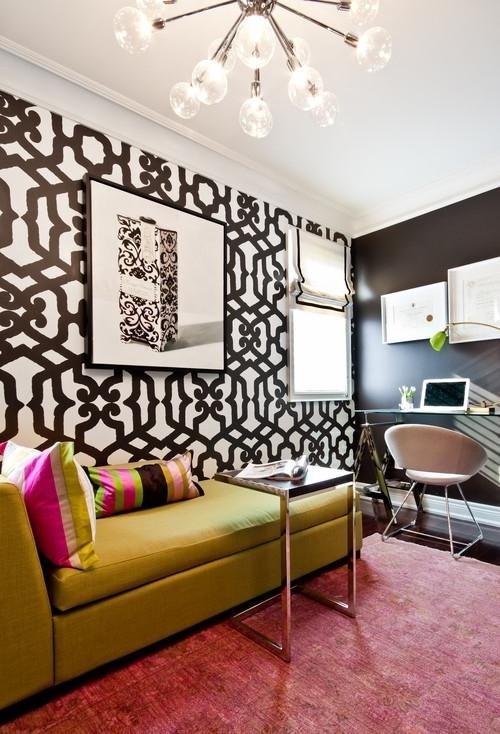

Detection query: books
[{"left": 235, "top": 455, "right": 309, "bottom": 481}]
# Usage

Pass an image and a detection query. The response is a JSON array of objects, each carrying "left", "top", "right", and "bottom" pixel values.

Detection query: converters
[{"left": 470, "top": 406, "right": 496, "bottom": 415}]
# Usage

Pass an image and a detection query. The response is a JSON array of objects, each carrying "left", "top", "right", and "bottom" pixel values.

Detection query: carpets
[{"left": 2, "top": 532, "right": 500, "bottom": 733}]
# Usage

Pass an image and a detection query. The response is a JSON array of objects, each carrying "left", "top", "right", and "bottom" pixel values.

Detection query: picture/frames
[
  {"left": 83, "top": 172, "right": 228, "bottom": 374},
  {"left": 380, "top": 255, "right": 500, "bottom": 346}
]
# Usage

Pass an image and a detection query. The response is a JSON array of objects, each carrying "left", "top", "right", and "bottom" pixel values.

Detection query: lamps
[
  {"left": 111, "top": 0, "right": 395, "bottom": 141},
  {"left": 428, "top": 321, "right": 500, "bottom": 351}
]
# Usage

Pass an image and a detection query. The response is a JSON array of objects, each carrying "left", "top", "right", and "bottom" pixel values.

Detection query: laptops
[{"left": 399, "top": 376, "right": 471, "bottom": 414}]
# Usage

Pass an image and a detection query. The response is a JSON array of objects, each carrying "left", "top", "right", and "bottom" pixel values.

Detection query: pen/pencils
[{"left": 398, "top": 384, "right": 417, "bottom": 399}]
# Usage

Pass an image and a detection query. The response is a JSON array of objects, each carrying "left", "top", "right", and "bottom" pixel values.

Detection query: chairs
[{"left": 381, "top": 422, "right": 489, "bottom": 559}]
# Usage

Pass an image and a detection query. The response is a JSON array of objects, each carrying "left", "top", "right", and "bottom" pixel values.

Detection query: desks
[
  {"left": 212, "top": 464, "right": 357, "bottom": 663},
  {"left": 353, "top": 405, "right": 499, "bottom": 526}
]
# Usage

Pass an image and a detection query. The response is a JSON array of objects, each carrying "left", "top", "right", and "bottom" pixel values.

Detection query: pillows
[{"left": 0, "top": 440, "right": 206, "bottom": 569}]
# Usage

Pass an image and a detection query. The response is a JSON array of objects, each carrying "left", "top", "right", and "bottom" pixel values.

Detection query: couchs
[{"left": 0, "top": 438, "right": 363, "bottom": 712}]
